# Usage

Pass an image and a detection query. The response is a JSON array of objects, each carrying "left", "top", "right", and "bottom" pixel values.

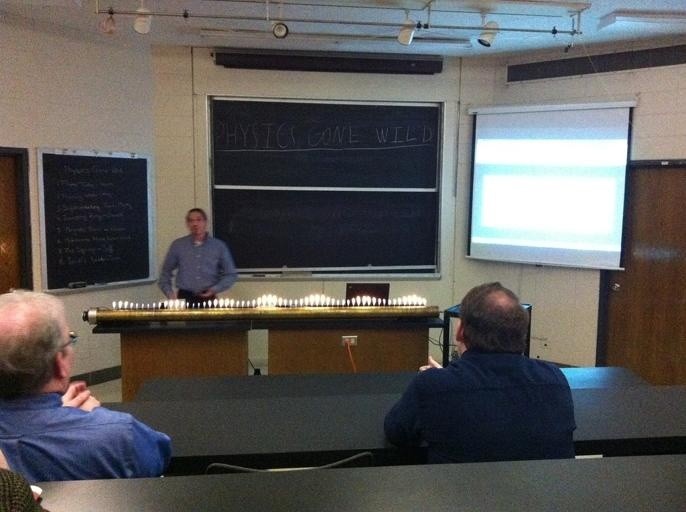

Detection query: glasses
[{"left": 60, "top": 330, "right": 81, "bottom": 352}]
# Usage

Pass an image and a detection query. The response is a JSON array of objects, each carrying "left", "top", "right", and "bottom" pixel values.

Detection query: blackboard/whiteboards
[
  {"left": 203, "top": 91, "right": 448, "bottom": 282},
  {"left": 36, "top": 144, "right": 158, "bottom": 296}
]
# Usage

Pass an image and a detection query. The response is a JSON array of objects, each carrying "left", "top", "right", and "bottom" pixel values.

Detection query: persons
[
  {"left": 1, "top": 288, "right": 172, "bottom": 482},
  {"left": 383, "top": 280, "right": 577, "bottom": 462},
  {"left": 0, "top": 449, "right": 47, "bottom": 511},
  {"left": 159, "top": 207, "right": 239, "bottom": 307}
]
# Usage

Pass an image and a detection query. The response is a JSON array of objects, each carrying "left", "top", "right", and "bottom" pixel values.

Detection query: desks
[{"left": 442, "top": 302, "right": 532, "bottom": 368}]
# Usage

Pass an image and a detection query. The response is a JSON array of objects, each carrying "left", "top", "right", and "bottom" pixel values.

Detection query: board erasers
[{"left": 68, "top": 281, "right": 88, "bottom": 290}]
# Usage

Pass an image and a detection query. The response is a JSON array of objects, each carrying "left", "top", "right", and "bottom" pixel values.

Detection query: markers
[{"left": 252, "top": 275, "right": 267, "bottom": 278}]
